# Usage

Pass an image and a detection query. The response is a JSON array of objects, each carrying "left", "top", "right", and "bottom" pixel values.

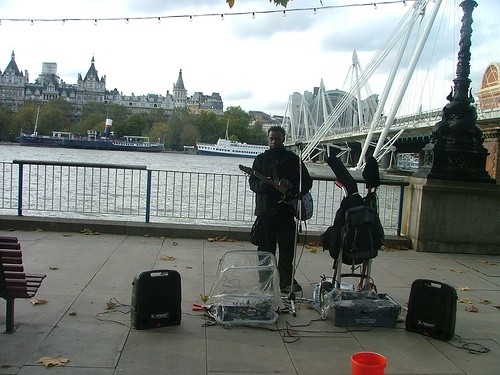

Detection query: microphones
[{"left": 295, "top": 140, "right": 302, "bottom": 146}]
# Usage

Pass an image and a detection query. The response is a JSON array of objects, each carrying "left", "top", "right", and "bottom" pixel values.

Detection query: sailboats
[{"left": 299, "top": 0, "right": 455, "bottom": 185}]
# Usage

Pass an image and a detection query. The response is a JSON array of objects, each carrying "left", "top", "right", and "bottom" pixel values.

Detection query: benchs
[{"left": 0, "top": 235, "right": 46, "bottom": 335}]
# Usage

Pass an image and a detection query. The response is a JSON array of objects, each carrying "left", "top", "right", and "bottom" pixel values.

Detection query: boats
[
  {"left": 19, "top": 104, "right": 167, "bottom": 153},
  {"left": 194, "top": 119, "right": 269, "bottom": 159}
]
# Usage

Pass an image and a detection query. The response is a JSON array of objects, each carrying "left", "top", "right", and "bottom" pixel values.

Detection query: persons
[{"left": 248, "top": 125, "right": 313, "bottom": 294}]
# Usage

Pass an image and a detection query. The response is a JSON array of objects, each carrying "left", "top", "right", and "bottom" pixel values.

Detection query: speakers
[
  {"left": 130, "top": 269, "right": 181, "bottom": 330},
  {"left": 406, "top": 279, "right": 458, "bottom": 340}
]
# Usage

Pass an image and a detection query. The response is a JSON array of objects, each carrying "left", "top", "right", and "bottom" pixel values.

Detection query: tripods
[{"left": 282, "top": 144, "right": 313, "bottom": 315}]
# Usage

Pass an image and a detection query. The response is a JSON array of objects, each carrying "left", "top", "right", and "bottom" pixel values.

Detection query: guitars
[{"left": 239, "top": 164, "right": 313, "bottom": 221}]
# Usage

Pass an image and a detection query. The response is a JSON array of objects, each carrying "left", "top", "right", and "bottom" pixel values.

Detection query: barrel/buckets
[{"left": 351, "top": 352, "right": 386, "bottom": 375}]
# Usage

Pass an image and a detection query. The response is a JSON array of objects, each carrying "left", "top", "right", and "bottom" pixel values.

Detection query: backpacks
[{"left": 344, "top": 204, "right": 379, "bottom": 254}]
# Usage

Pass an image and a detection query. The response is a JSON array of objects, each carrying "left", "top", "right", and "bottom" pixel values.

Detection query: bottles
[{"left": 313, "top": 284, "right": 320, "bottom": 306}]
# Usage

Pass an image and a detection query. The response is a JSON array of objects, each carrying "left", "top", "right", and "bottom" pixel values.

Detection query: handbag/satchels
[
  {"left": 292, "top": 192, "right": 313, "bottom": 220},
  {"left": 248, "top": 218, "right": 261, "bottom": 247}
]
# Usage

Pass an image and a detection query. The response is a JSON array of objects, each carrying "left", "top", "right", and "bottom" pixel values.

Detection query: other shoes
[{"left": 281, "top": 284, "right": 301, "bottom": 293}]
